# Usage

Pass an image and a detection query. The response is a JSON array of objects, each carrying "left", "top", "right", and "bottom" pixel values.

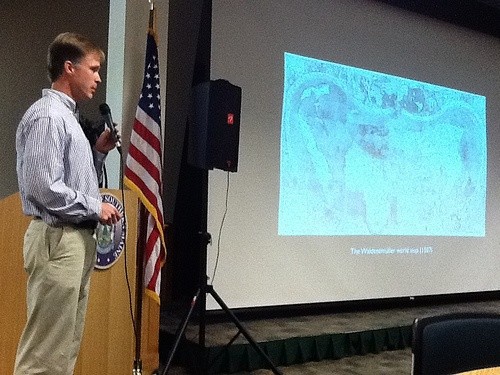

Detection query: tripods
[{"left": 160, "top": 173, "right": 283, "bottom": 375}]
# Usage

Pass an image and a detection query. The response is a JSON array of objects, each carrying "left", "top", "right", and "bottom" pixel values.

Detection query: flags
[{"left": 123, "top": 28, "right": 166, "bottom": 307}]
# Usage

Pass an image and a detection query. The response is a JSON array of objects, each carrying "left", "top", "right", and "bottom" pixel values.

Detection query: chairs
[{"left": 411, "top": 312, "right": 500, "bottom": 375}]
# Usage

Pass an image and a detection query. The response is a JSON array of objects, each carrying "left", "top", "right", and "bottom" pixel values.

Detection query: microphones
[{"left": 98, "top": 103, "right": 122, "bottom": 154}]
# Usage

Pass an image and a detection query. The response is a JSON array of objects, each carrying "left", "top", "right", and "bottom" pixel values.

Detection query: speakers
[{"left": 186, "top": 80, "right": 242, "bottom": 172}]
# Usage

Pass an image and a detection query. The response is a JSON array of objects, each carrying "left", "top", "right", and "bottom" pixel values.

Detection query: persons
[{"left": 13, "top": 33, "right": 123, "bottom": 375}]
